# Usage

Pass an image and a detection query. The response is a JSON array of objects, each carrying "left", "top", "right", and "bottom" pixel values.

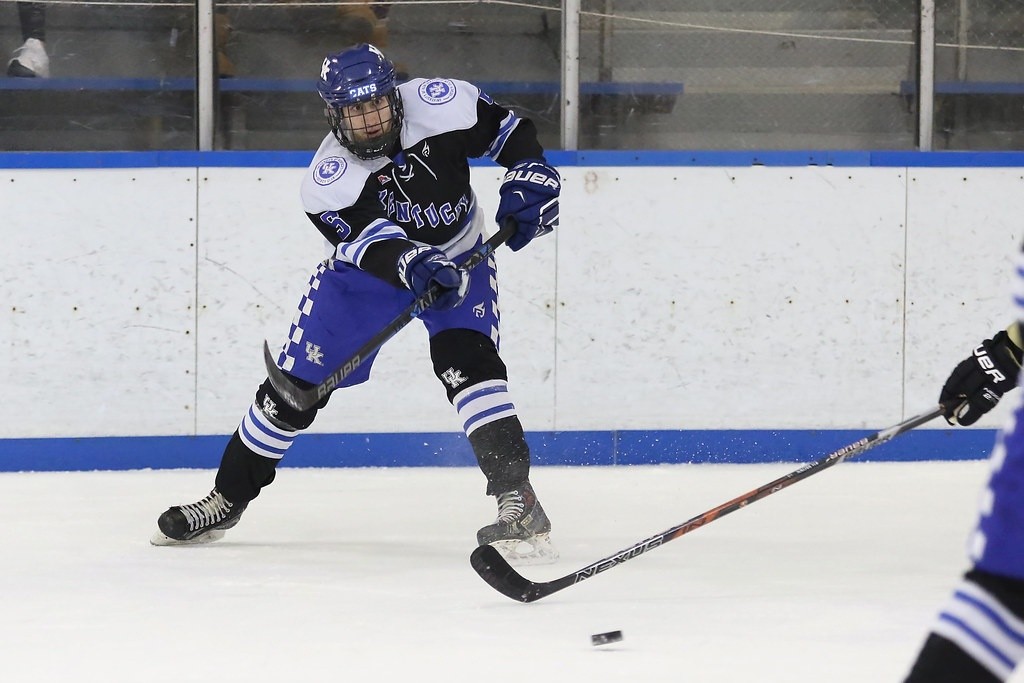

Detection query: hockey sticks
[
  {"left": 468, "top": 398, "right": 965, "bottom": 602},
  {"left": 263, "top": 221, "right": 517, "bottom": 411}
]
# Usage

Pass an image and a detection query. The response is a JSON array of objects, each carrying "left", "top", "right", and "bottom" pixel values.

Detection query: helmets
[{"left": 315, "top": 43, "right": 404, "bottom": 160}]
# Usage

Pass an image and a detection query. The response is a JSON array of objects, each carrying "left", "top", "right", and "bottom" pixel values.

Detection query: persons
[
  {"left": 7, "top": 1, "right": 49, "bottom": 79},
  {"left": 900, "top": 236, "right": 1024, "bottom": 683},
  {"left": 146, "top": 42, "right": 562, "bottom": 570}
]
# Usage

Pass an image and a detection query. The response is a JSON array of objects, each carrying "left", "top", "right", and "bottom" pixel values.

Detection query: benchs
[
  {"left": 0, "top": 1, "right": 684, "bottom": 150},
  {"left": 900, "top": 1, "right": 1023, "bottom": 148}
]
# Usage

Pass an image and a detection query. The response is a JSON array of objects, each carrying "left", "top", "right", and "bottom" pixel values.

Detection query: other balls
[{"left": 590, "top": 629, "right": 624, "bottom": 647}]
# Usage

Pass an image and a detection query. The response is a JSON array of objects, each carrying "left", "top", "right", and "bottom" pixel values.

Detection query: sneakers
[
  {"left": 149, "top": 485, "right": 249, "bottom": 546},
  {"left": 476, "top": 480, "right": 559, "bottom": 567},
  {"left": 5, "top": 38, "right": 50, "bottom": 78}
]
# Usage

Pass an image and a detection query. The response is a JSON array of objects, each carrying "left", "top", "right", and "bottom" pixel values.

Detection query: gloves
[
  {"left": 496, "top": 159, "right": 562, "bottom": 252},
  {"left": 397, "top": 245, "right": 471, "bottom": 311},
  {"left": 939, "top": 330, "right": 1024, "bottom": 427}
]
